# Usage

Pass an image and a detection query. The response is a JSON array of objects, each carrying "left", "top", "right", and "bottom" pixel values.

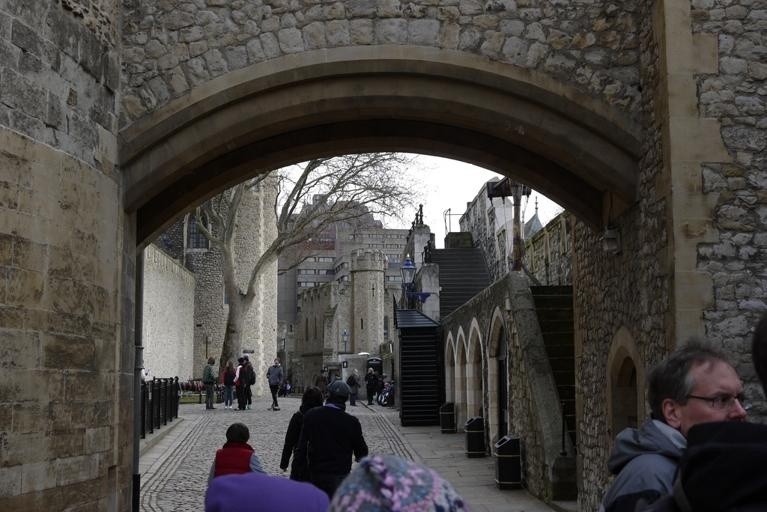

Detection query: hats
[{"left": 325, "top": 379, "right": 353, "bottom": 401}]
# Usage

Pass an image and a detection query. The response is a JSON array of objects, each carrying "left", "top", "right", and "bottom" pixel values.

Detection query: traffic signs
[{"left": 399, "top": 253, "right": 417, "bottom": 309}]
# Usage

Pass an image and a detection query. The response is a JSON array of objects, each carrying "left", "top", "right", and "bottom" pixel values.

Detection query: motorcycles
[
  {"left": 278, "top": 384, "right": 292, "bottom": 397},
  {"left": 377, "top": 381, "right": 394, "bottom": 407}
]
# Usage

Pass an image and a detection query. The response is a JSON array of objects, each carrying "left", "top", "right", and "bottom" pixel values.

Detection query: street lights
[{"left": 342, "top": 329, "right": 349, "bottom": 352}]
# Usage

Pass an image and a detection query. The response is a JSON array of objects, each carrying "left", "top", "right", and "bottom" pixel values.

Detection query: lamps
[
  {"left": 599, "top": 226, "right": 623, "bottom": 256},
  {"left": 400, "top": 252, "right": 438, "bottom": 303}
]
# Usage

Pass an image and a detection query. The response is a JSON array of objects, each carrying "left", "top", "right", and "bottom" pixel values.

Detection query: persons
[
  {"left": 266, "top": 356, "right": 283, "bottom": 410},
  {"left": 376, "top": 373, "right": 386, "bottom": 401},
  {"left": 292, "top": 380, "right": 368, "bottom": 498},
  {"left": 279, "top": 386, "right": 323, "bottom": 481},
  {"left": 315, "top": 368, "right": 327, "bottom": 402},
  {"left": 233, "top": 357, "right": 248, "bottom": 410},
  {"left": 207, "top": 421, "right": 267, "bottom": 490},
  {"left": 598, "top": 334, "right": 747, "bottom": 512},
  {"left": 364, "top": 367, "right": 377, "bottom": 406},
  {"left": 349, "top": 368, "right": 362, "bottom": 406},
  {"left": 201, "top": 355, "right": 219, "bottom": 409},
  {"left": 645, "top": 306, "right": 766, "bottom": 512},
  {"left": 332, "top": 370, "right": 343, "bottom": 381},
  {"left": 243, "top": 353, "right": 255, "bottom": 408},
  {"left": 223, "top": 360, "right": 235, "bottom": 408},
  {"left": 283, "top": 379, "right": 291, "bottom": 397}
]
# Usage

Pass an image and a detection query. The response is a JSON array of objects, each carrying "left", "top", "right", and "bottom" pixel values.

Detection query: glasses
[{"left": 685, "top": 390, "right": 756, "bottom": 414}]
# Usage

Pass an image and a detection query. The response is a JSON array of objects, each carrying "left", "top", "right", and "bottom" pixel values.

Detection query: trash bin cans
[
  {"left": 494, "top": 436, "right": 521, "bottom": 489},
  {"left": 440, "top": 402, "right": 457, "bottom": 433},
  {"left": 463, "top": 417, "right": 486, "bottom": 458}
]
